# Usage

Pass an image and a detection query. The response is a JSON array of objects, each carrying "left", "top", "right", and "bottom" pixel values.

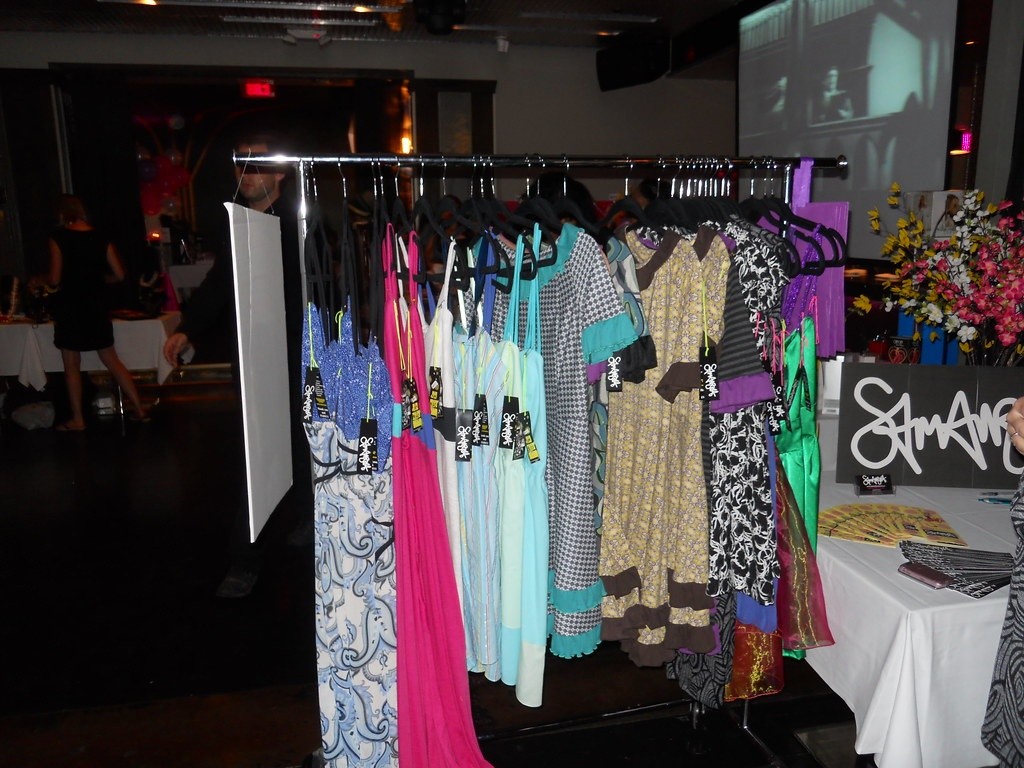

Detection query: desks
[
  {"left": 0, "top": 309, "right": 195, "bottom": 416},
  {"left": 164, "top": 258, "right": 215, "bottom": 304},
  {"left": 803, "top": 414, "right": 1024, "bottom": 768}
]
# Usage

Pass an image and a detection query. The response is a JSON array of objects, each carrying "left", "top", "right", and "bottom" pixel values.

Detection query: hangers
[
  {"left": 296, "top": 153, "right": 329, "bottom": 251},
  {"left": 369, "top": 154, "right": 847, "bottom": 293},
  {"left": 336, "top": 154, "right": 349, "bottom": 243}
]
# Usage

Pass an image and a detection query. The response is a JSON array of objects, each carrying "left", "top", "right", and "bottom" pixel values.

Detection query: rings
[{"left": 1011, "top": 433, "right": 1018, "bottom": 438}]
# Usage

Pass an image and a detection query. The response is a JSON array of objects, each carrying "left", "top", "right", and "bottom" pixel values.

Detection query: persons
[
  {"left": 27, "top": 194, "right": 151, "bottom": 431},
  {"left": 163, "top": 134, "right": 339, "bottom": 548},
  {"left": 980, "top": 393, "right": 1024, "bottom": 768}
]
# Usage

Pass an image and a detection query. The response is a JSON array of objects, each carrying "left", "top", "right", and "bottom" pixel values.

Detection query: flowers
[{"left": 853, "top": 181, "right": 1024, "bottom": 365}]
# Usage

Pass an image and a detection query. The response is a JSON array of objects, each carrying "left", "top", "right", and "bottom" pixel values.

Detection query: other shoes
[
  {"left": 55, "top": 418, "right": 86, "bottom": 430},
  {"left": 126, "top": 408, "right": 152, "bottom": 423}
]
